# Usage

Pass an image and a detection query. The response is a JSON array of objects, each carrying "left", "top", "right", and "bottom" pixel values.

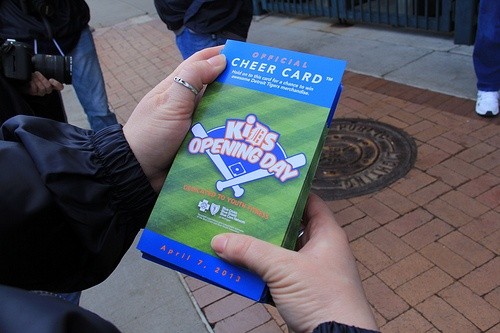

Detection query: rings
[
  {"left": 174, "top": 77, "right": 198, "bottom": 95},
  {"left": 298, "top": 231, "right": 304, "bottom": 238}
]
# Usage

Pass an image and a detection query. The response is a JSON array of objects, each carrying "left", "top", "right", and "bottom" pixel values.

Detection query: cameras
[{"left": 2, "top": 39, "right": 74, "bottom": 86}]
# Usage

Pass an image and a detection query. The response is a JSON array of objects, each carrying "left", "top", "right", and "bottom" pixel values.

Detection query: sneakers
[{"left": 475, "top": 88, "right": 499, "bottom": 116}]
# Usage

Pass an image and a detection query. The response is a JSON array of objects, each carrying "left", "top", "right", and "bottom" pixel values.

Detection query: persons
[
  {"left": 0, "top": 0, "right": 118, "bottom": 134},
  {"left": 154, "top": 0, "right": 254, "bottom": 60},
  {"left": 0, "top": 44, "right": 382, "bottom": 333},
  {"left": 473, "top": 0, "right": 500, "bottom": 117}
]
